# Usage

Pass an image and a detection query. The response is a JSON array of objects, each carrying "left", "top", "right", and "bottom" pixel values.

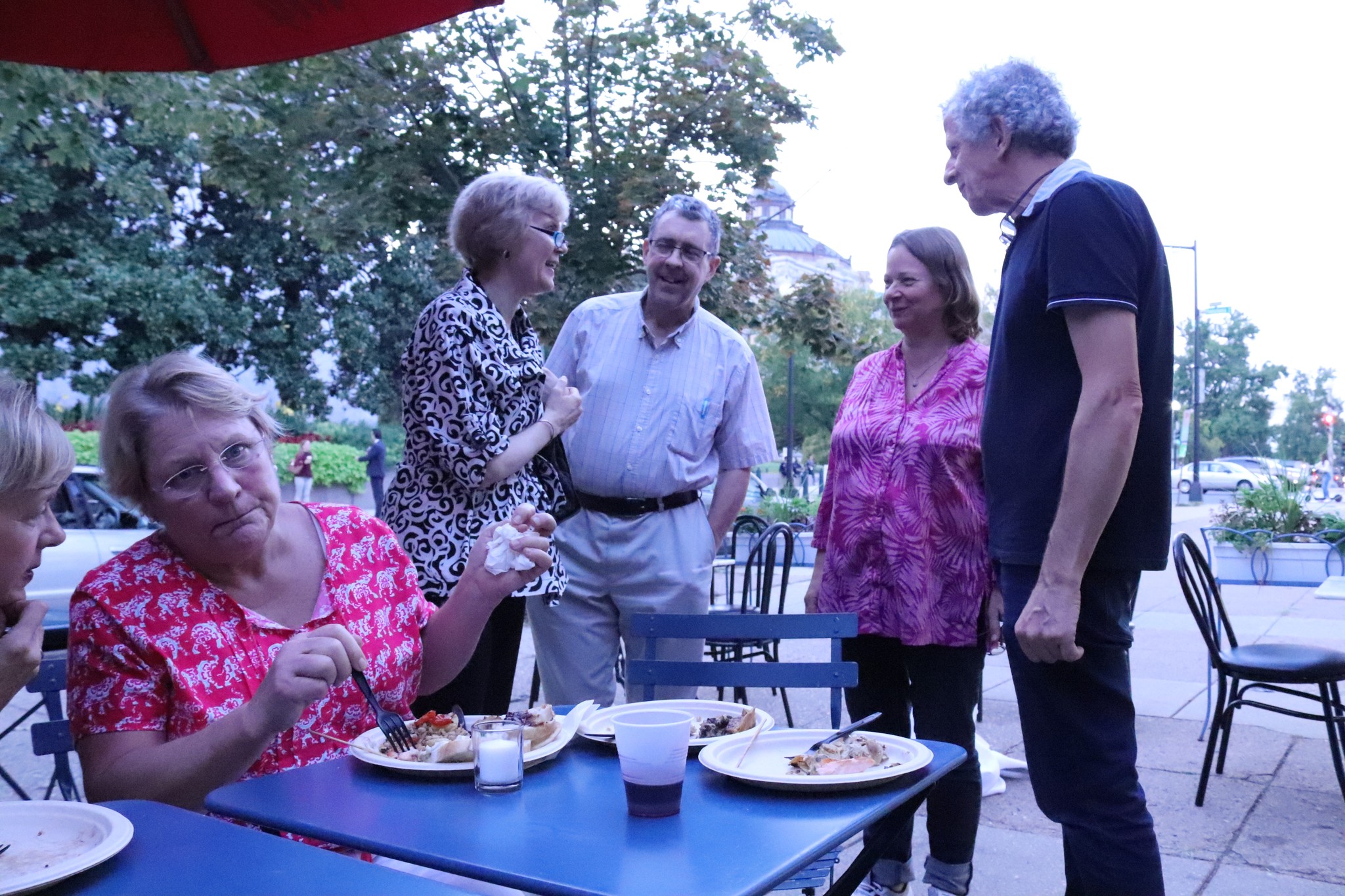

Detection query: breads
[
  {"left": 483, "top": 715, "right": 555, "bottom": 745},
  {"left": 431, "top": 737, "right": 532, "bottom": 762}
]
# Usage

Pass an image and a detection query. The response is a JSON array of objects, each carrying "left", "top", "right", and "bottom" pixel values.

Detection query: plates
[
  {"left": 0, "top": 801, "right": 134, "bottom": 896},
  {"left": 698, "top": 729, "right": 934, "bottom": 792},
  {"left": 552, "top": 705, "right": 605, "bottom": 715},
  {"left": 576, "top": 699, "right": 775, "bottom": 753},
  {"left": 349, "top": 715, "right": 566, "bottom": 778}
]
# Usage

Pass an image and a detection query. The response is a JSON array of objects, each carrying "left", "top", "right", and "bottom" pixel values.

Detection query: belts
[{"left": 573, "top": 486, "right": 701, "bottom": 516}]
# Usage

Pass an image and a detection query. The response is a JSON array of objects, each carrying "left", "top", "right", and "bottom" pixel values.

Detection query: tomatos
[{"left": 408, "top": 710, "right": 454, "bottom": 742}]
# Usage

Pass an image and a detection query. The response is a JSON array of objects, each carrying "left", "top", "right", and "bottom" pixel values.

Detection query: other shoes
[{"left": 1324, "top": 498, "right": 1330, "bottom": 502}]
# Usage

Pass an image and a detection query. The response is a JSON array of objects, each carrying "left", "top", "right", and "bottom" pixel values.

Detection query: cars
[
  {"left": 1, "top": 466, "right": 165, "bottom": 632},
  {"left": 1171, "top": 456, "right": 1345, "bottom": 495},
  {"left": 699, "top": 468, "right": 804, "bottom": 534}
]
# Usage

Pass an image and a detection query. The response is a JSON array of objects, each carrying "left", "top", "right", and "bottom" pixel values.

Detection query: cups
[
  {"left": 610, "top": 709, "right": 695, "bottom": 818},
  {"left": 471, "top": 720, "right": 523, "bottom": 794}
]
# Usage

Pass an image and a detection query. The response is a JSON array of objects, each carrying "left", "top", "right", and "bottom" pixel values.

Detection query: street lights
[{"left": 1171, "top": 401, "right": 1181, "bottom": 470}]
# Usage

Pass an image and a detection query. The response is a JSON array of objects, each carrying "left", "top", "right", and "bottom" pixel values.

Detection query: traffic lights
[{"left": 1326, "top": 414, "right": 1334, "bottom": 432}]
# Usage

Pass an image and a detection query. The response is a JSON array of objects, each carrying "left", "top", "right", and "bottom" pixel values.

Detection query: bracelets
[{"left": 533, "top": 420, "right": 554, "bottom": 446}]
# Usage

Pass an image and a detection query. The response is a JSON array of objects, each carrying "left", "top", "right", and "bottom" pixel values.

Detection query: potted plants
[
  {"left": 1210, "top": 441, "right": 1345, "bottom": 579},
  {"left": 731, "top": 487, "right": 816, "bottom": 569}
]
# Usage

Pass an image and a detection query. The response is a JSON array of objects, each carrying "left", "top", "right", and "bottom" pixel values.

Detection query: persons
[
  {"left": 780, "top": 454, "right": 815, "bottom": 487},
  {"left": 0, "top": 378, "right": 75, "bottom": 710},
  {"left": 805, "top": 226, "right": 1006, "bottom": 896},
  {"left": 294, "top": 439, "right": 314, "bottom": 504},
  {"left": 943, "top": 60, "right": 1175, "bottom": 896},
  {"left": 526, "top": 193, "right": 778, "bottom": 710},
  {"left": 380, "top": 168, "right": 583, "bottom": 717},
  {"left": 1314, "top": 453, "right": 1332, "bottom": 498},
  {"left": 355, "top": 429, "right": 386, "bottom": 519},
  {"left": 65, "top": 350, "right": 556, "bottom": 896}
]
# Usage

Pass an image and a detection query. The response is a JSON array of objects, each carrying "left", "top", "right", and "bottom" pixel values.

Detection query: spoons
[{"left": 450, "top": 704, "right": 471, "bottom": 738}]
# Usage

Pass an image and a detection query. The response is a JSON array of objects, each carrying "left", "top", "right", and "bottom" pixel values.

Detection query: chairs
[
  {"left": 1170, "top": 530, "right": 1345, "bottom": 809},
  {"left": 22, "top": 658, "right": 93, "bottom": 797},
  {"left": 624, "top": 609, "right": 861, "bottom": 732},
  {"left": 716, "top": 512, "right": 804, "bottom": 730}
]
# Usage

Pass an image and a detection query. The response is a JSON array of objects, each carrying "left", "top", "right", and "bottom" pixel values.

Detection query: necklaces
[{"left": 906, "top": 352, "right": 946, "bottom": 387}]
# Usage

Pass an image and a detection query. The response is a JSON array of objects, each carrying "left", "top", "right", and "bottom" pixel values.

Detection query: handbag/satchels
[
  {"left": 288, "top": 460, "right": 304, "bottom": 474},
  {"left": 537, "top": 434, "right": 581, "bottom": 522}
]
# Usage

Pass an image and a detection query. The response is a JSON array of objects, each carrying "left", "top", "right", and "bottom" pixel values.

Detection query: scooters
[{"left": 1305, "top": 468, "right": 1342, "bottom": 503}]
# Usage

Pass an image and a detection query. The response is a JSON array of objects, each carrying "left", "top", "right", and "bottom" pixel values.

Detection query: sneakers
[{"left": 851, "top": 877, "right": 914, "bottom": 896}]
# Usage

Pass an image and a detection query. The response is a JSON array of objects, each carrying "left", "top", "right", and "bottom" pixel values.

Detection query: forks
[
  {"left": 351, "top": 666, "right": 417, "bottom": 753},
  {"left": 784, "top": 712, "right": 883, "bottom": 760}
]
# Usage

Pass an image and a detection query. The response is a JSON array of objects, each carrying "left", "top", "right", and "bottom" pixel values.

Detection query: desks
[
  {"left": 0, "top": 797, "right": 484, "bottom": 896},
  {"left": 203, "top": 700, "right": 969, "bottom": 896}
]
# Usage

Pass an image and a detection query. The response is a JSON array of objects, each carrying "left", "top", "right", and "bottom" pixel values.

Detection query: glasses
[
  {"left": 649, "top": 239, "right": 712, "bottom": 265},
  {"left": 150, "top": 437, "right": 265, "bottom": 499},
  {"left": 529, "top": 225, "right": 569, "bottom": 248}
]
